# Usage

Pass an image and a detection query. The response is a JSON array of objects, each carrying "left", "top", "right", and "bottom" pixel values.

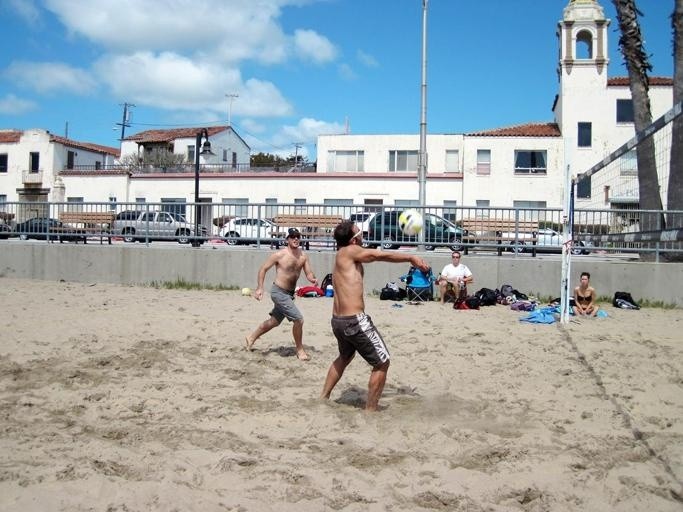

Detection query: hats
[{"left": 286, "top": 228, "right": 301, "bottom": 239}]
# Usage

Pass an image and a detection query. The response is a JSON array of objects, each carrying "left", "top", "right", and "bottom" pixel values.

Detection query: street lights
[{"left": 190, "top": 127, "right": 217, "bottom": 247}]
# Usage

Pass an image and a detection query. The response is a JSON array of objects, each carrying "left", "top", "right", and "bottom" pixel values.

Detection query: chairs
[{"left": 404, "top": 264, "right": 434, "bottom": 303}]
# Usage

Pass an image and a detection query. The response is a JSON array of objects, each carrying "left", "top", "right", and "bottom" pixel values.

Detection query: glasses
[
  {"left": 452, "top": 256, "right": 459, "bottom": 259},
  {"left": 350, "top": 229, "right": 362, "bottom": 241},
  {"left": 290, "top": 235, "right": 300, "bottom": 239}
]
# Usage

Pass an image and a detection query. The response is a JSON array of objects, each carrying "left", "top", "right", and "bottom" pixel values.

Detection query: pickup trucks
[{"left": 495, "top": 228, "right": 593, "bottom": 255}]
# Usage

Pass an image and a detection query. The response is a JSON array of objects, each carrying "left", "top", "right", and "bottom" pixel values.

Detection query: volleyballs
[
  {"left": 243, "top": 288, "right": 252, "bottom": 296},
  {"left": 400, "top": 210, "right": 421, "bottom": 236}
]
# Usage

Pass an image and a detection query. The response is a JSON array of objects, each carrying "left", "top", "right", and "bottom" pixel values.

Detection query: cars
[
  {"left": 0, "top": 210, "right": 207, "bottom": 244},
  {"left": 219, "top": 217, "right": 306, "bottom": 245},
  {"left": 349, "top": 211, "right": 479, "bottom": 251}
]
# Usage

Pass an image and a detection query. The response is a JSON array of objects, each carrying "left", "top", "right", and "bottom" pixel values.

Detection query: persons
[
  {"left": 319, "top": 221, "right": 430, "bottom": 412},
  {"left": 439, "top": 251, "right": 473, "bottom": 305},
  {"left": 572, "top": 271, "right": 599, "bottom": 317},
  {"left": 246, "top": 228, "right": 318, "bottom": 361}
]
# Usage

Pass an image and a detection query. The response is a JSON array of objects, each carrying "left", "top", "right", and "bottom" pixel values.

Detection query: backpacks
[
  {"left": 465, "top": 285, "right": 537, "bottom": 311},
  {"left": 321, "top": 273, "right": 334, "bottom": 291},
  {"left": 612, "top": 291, "right": 640, "bottom": 310}
]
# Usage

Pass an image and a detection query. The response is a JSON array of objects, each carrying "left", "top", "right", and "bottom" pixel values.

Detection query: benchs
[
  {"left": 49, "top": 211, "right": 114, "bottom": 245},
  {"left": 267, "top": 214, "right": 343, "bottom": 251},
  {"left": 461, "top": 217, "right": 539, "bottom": 257}
]
